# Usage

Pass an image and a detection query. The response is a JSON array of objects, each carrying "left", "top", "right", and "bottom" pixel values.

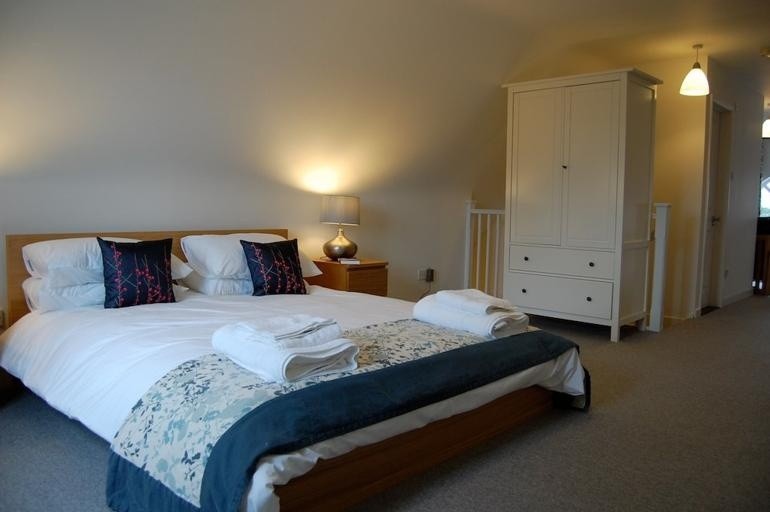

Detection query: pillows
[
  {"left": 239, "top": 239, "right": 307, "bottom": 296},
  {"left": 96, "top": 236, "right": 177, "bottom": 309}
]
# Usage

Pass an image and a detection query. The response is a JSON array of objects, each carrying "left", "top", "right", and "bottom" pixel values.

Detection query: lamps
[
  {"left": 762, "top": 117, "right": 770, "bottom": 139},
  {"left": 319, "top": 196, "right": 362, "bottom": 261},
  {"left": 679, "top": 44, "right": 710, "bottom": 97}
]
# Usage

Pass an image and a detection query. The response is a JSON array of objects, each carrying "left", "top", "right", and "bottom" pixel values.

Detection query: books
[{"left": 338, "top": 258, "right": 361, "bottom": 265}]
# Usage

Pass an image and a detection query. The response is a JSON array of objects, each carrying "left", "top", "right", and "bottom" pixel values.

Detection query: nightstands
[{"left": 305, "top": 255, "right": 389, "bottom": 298}]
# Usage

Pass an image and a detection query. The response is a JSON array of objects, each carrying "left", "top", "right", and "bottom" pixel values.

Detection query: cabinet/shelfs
[{"left": 501, "top": 67, "right": 664, "bottom": 344}]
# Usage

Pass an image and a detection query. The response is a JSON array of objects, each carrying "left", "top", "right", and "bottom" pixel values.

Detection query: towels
[
  {"left": 413, "top": 289, "right": 530, "bottom": 339},
  {"left": 214, "top": 318, "right": 360, "bottom": 382}
]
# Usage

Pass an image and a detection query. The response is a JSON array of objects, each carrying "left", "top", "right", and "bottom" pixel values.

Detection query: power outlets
[{"left": 418, "top": 269, "right": 427, "bottom": 281}]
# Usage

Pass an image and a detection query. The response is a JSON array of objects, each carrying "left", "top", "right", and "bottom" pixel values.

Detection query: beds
[{"left": 0, "top": 229, "right": 591, "bottom": 512}]
